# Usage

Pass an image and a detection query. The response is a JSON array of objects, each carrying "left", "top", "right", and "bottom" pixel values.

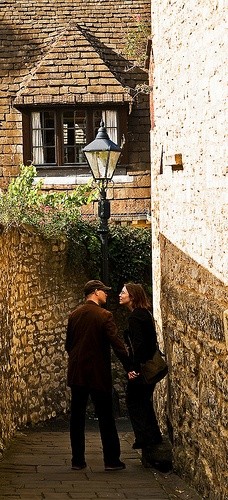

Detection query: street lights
[{"left": 80, "top": 118, "right": 125, "bottom": 419}]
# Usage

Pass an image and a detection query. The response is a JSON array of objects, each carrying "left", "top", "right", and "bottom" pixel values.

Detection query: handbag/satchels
[{"left": 140, "top": 350, "right": 168, "bottom": 385}]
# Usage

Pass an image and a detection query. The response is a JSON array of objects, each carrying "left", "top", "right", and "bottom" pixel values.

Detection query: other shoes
[
  {"left": 152, "top": 432, "right": 163, "bottom": 442},
  {"left": 105, "top": 460, "right": 126, "bottom": 469},
  {"left": 71, "top": 463, "right": 86, "bottom": 468},
  {"left": 132, "top": 437, "right": 154, "bottom": 449}
]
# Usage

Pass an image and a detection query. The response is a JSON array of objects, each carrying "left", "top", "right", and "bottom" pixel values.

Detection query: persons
[
  {"left": 119, "top": 281, "right": 163, "bottom": 468},
  {"left": 64, "top": 281, "right": 130, "bottom": 469}
]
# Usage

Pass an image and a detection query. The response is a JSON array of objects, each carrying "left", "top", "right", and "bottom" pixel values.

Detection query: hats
[{"left": 85, "top": 280, "right": 112, "bottom": 294}]
